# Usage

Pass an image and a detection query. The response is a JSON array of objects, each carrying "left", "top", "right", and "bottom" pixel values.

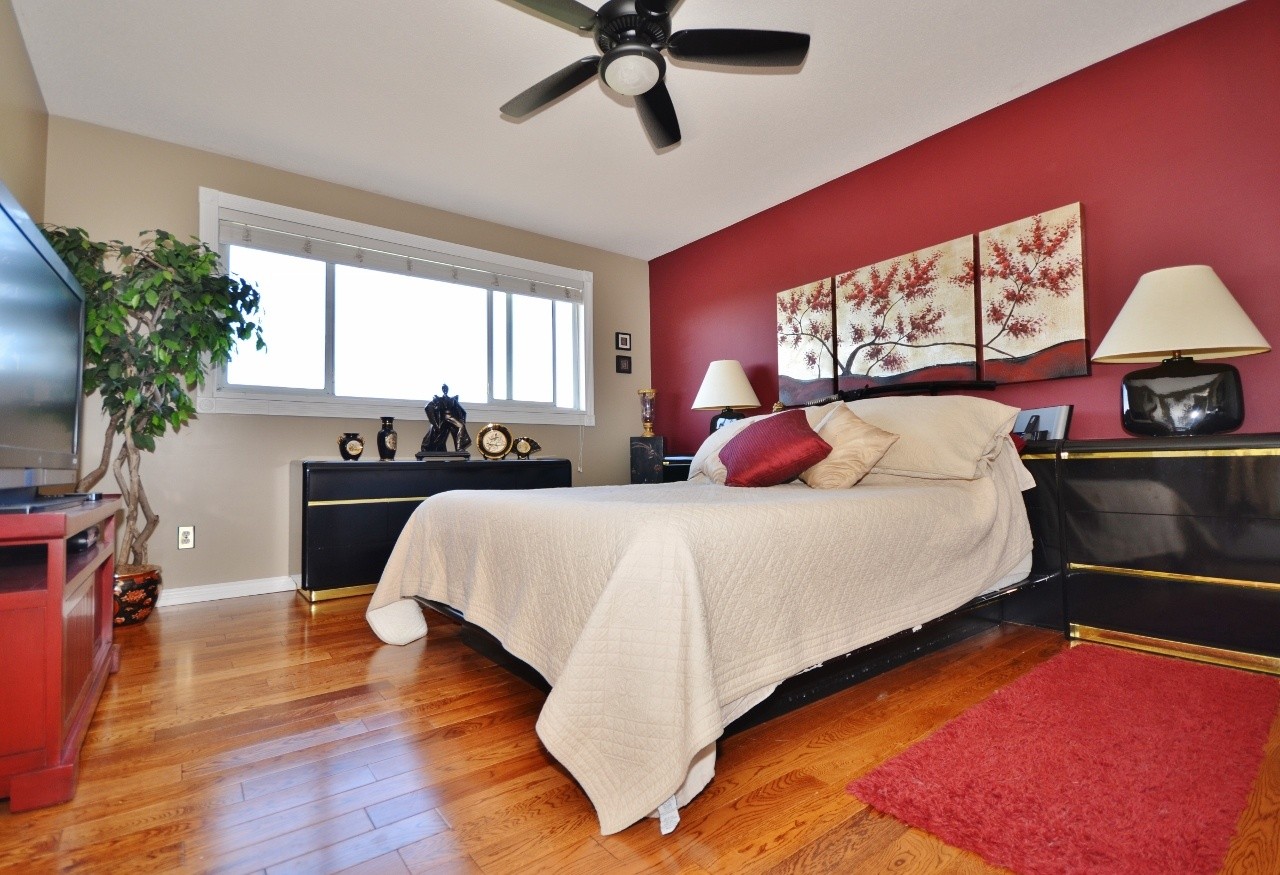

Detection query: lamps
[
  {"left": 691, "top": 359, "right": 762, "bottom": 438},
  {"left": 1090, "top": 264, "right": 1274, "bottom": 439}
]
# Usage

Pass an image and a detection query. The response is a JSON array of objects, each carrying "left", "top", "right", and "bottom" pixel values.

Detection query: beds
[{"left": 368, "top": 379, "right": 1065, "bottom": 837}]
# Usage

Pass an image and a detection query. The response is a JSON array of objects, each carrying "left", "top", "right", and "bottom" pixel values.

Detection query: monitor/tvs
[{"left": 0, "top": 180, "right": 88, "bottom": 513}]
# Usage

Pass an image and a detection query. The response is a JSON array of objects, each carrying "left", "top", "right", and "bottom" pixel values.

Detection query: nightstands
[
  {"left": 662, "top": 454, "right": 694, "bottom": 484},
  {"left": 1061, "top": 432, "right": 1280, "bottom": 677}
]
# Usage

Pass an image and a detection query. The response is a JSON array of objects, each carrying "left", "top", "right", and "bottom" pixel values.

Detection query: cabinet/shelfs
[
  {"left": 0, "top": 493, "right": 125, "bottom": 814},
  {"left": 286, "top": 458, "right": 573, "bottom": 605}
]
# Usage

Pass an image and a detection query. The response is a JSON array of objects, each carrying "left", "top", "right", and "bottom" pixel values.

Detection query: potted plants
[{"left": 35, "top": 223, "right": 267, "bottom": 628}]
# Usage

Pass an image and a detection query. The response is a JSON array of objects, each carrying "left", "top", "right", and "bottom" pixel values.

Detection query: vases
[
  {"left": 336, "top": 432, "right": 367, "bottom": 461},
  {"left": 377, "top": 417, "right": 397, "bottom": 461}
]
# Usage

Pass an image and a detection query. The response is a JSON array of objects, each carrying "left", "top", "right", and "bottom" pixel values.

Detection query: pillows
[
  {"left": 795, "top": 400, "right": 901, "bottom": 490},
  {"left": 718, "top": 408, "right": 833, "bottom": 489}
]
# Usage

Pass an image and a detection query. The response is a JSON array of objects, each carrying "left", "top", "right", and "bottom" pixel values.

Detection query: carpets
[{"left": 839, "top": 644, "right": 1280, "bottom": 875}]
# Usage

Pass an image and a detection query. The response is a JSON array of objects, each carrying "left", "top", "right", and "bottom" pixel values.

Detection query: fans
[{"left": 498, "top": 0, "right": 811, "bottom": 150}]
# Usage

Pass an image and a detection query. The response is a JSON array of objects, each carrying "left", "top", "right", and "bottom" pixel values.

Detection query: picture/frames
[
  {"left": 615, "top": 332, "right": 631, "bottom": 351},
  {"left": 616, "top": 355, "right": 632, "bottom": 374}
]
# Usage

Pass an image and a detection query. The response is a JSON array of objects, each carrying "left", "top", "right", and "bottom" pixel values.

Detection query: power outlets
[{"left": 178, "top": 525, "right": 195, "bottom": 550}]
